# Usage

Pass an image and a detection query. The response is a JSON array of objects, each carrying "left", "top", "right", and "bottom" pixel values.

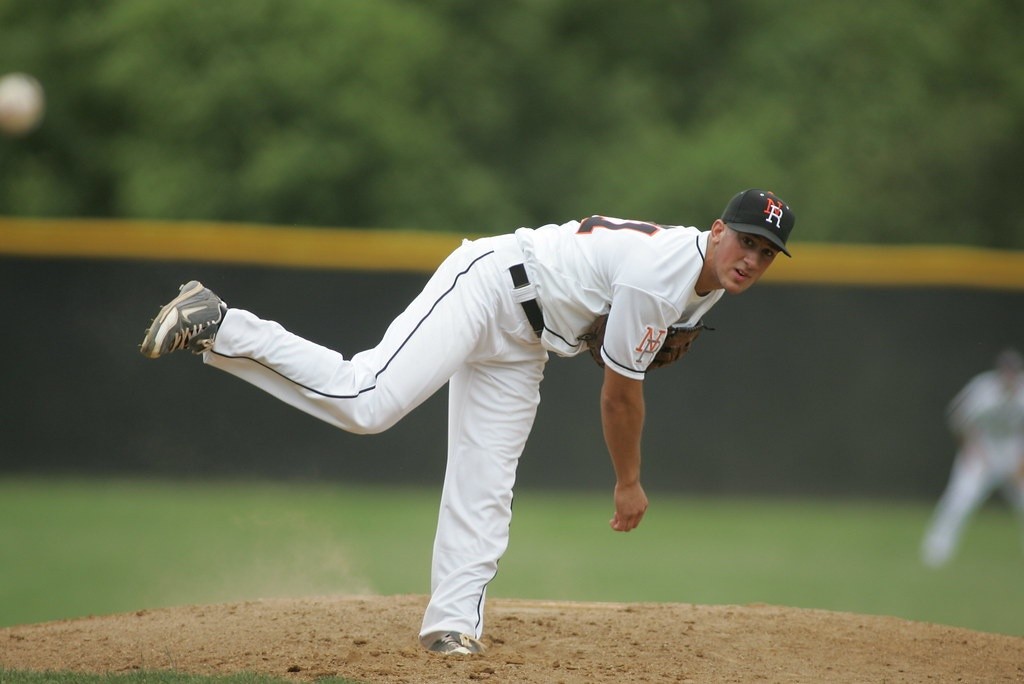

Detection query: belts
[{"left": 508, "top": 263, "right": 545, "bottom": 339}]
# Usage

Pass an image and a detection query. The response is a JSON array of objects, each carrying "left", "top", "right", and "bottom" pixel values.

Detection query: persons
[
  {"left": 926, "top": 350, "right": 1024, "bottom": 572},
  {"left": 138, "top": 186, "right": 794, "bottom": 657}
]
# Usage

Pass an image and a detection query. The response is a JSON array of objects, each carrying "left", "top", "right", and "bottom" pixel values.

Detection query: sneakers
[
  {"left": 139, "top": 281, "right": 227, "bottom": 359},
  {"left": 427, "top": 631, "right": 488, "bottom": 659}
]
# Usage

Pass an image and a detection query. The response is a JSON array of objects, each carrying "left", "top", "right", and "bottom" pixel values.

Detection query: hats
[{"left": 721, "top": 188, "right": 795, "bottom": 258}]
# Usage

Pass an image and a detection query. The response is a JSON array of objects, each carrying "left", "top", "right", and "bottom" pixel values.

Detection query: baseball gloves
[{"left": 586, "top": 313, "right": 717, "bottom": 375}]
